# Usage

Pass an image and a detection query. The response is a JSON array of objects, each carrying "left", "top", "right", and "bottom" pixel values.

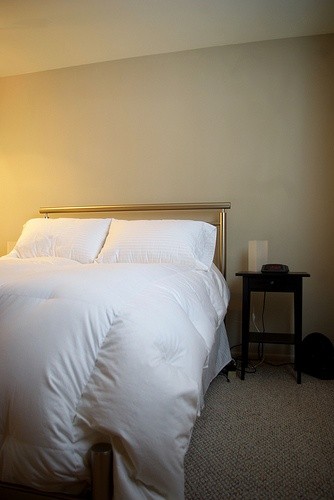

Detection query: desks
[{"left": 235, "top": 271, "right": 311, "bottom": 384}]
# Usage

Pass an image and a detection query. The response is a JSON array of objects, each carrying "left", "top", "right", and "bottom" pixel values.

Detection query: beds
[{"left": 0, "top": 202, "right": 232, "bottom": 500}]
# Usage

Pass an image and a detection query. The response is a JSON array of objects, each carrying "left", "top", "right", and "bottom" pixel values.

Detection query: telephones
[{"left": 262, "top": 263, "right": 289, "bottom": 273}]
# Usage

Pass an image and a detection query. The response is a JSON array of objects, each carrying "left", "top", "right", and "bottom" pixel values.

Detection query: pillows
[
  {"left": 94, "top": 218, "right": 217, "bottom": 271},
  {"left": 2, "top": 218, "right": 112, "bottom": 263}
]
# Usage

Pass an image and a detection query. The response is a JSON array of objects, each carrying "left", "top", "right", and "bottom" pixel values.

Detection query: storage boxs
[{"left": 248, "top": 240, "right": 268, "bottom": 272}]
